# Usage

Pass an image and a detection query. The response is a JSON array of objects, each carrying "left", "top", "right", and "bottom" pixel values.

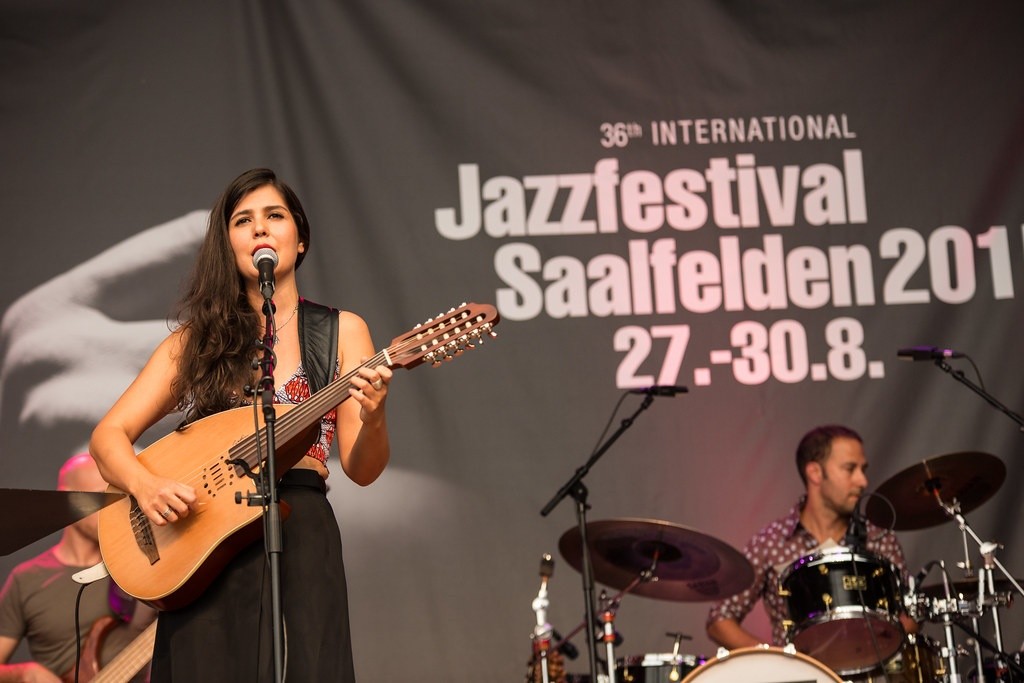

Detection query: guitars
[
  {"left": 60, "top": 610, "right": 158, "bottom": 683},
  {"left": 92, "top": 304, "right": 502, "bottom": 612}
]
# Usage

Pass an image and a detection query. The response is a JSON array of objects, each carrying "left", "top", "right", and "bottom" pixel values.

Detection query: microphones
[
  {"left": 628, "top": 385, "right": 690, "bottom": 398},
  {"left": 895, "top": 346, "right": 967, "bottom": 362},
  {"left": 253, "top": 247, "right": 278, "bottom": 302},
  {"left": 915, "top": 560, "right": 938, "bottom": 587},
  {"left": 593, "top": 613, "right": 625, "bottom": 647},
  {"left": 550, "top": 629, "right": 581, "bottom": 660}
]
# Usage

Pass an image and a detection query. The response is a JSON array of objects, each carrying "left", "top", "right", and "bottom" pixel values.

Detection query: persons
[
  {"left": 706, "top": 424, "right": 923, "bottom": 653},
  {"left": 0, "top": 454, "right": 158, "bottom": 682},
  {"left": 89, "top": 168, "right": 393, "bottom": 682}
]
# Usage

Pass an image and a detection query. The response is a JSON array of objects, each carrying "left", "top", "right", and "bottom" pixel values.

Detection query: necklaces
[{"left": 257, "top": 305, "right": 298, "bottom": 343}]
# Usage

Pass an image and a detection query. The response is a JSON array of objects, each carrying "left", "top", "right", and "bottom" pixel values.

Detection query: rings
[
  {"left": 372, "top": 377, "right": 383, "bottom": 390},
  {"left": 163, "top": 508, "right": 172, "bottom": 518}
]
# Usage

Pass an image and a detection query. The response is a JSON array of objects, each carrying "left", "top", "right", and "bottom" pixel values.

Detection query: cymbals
[
  {"left": 866, "top": 450, "right": 1004, "bottom": 533},
  {"left": 555, "top": 517, "right": 755, "bottom": 606}
]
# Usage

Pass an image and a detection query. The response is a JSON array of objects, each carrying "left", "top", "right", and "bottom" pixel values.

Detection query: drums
[
  {"left": 609, "top": 653, "right": 707, "bottom": 683},
  {"left": 681, "top": 647, "right": 843, "bottom": 683},
  {"left": 776, "top": 549, "right": 907, "bottom": 677}
]
